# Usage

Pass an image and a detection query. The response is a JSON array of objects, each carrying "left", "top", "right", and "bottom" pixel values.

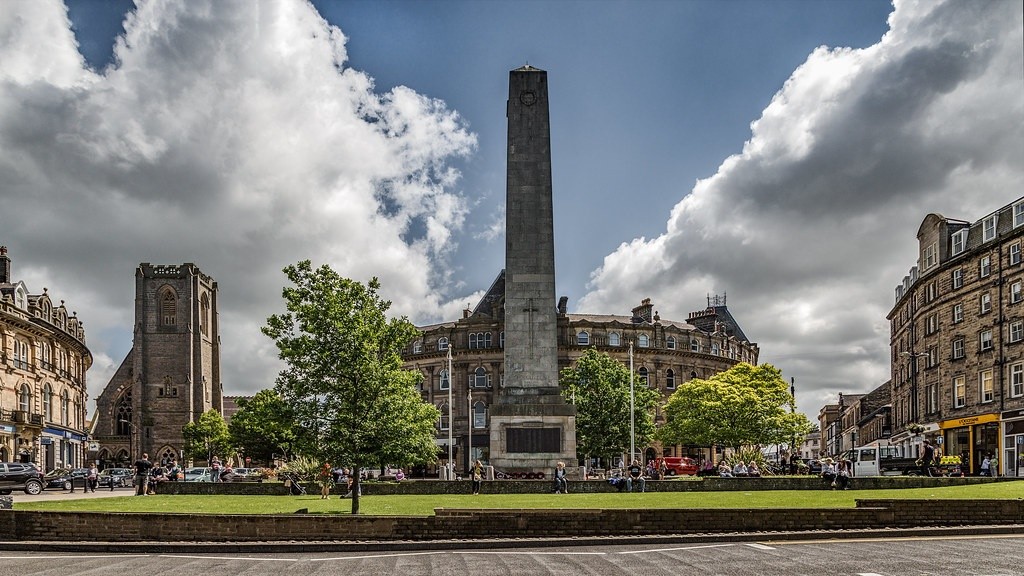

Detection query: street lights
[
  {"left": 467, "top": 389, "right": 473, "bottom": 480},
  {"left": 900, "top": 351, "right": 930, "bottom": 458}
]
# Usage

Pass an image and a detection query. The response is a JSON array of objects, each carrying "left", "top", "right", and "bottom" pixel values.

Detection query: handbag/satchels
[{"left": 474, "top": 472, "right": 481, "bottom": 482}]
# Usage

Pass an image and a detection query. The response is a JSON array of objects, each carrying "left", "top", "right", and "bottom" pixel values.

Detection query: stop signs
[{"left": 246, "top": 457, "right": 251, "bottom": 463}]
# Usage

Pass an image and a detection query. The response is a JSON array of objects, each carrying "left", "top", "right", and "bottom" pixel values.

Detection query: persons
[
  {"left": 626, "top": 459, "right": 645, "bottom": 492},
  {"left": 395, "top": 469, "right": 404, "bottom": 483},
  {"left": 319, "top": 464, "right": 361, "bottom": 499},
  {"left": 981, "top": 455, "right": 998, "bottom": 477},
  {"left": 814, "top": 456, "right": 850, "bottom": 490},
  {"left": 133, "top": 453, "right": 152, "bottom": 496},
  {"left": 695, "top": 458, "right": 760, "bottom": 478},
  {"left": 645, "top": 457, "right": 668, "bottom": 479},
  {"left": 618, "top": 459, "right": 624, "bottom": 469},
  {"left": 210, "top": 456, "right": 232, "bottom": 482},
  {"left": 554, "top": 462, "right": 568, "bottom": 494},
  {"left": 87, "top": 462, "right": 98, "bottom": 492},
  {"left": 780, "top": 453, "right": 799, "bottom": 475},
  {"left": 468, "top": 460, "right": 485, "bottom": 495},
  {"left": 915, "top": 439, "right": 934, "bottom": 477},
  {"left": 150, "top": 461, "right": 181, "bottom": 483}
]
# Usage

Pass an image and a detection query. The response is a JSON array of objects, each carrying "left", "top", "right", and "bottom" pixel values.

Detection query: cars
[
  {"left": 0, "top": 461, "right": 47, "bottom": 494},
  {"left": 593, "top": 469, "right": 604, "bottom": 477},
  {"left": 177, "top": 467, "right": 213, "bottom": 482},
  {"left": 97, "top": 468, "right": 134, "bottom": 487},
  {"left": 389, "top": 465, "right": 403, "bottom": 475},
  {"left": 784, "top": 459, "right": 822, "bottom": 475},
  {"left": 234, "top": 467, "right": 260, "bottom": 475},
  {"left": 132, "top": 467, "right": 170, "bottom": 487},
  {"left": 44, "top": 468, "right": 91, "bottom": 490},
  {"left": 359, "top": 466, "right": 382, "bottom": 479}
]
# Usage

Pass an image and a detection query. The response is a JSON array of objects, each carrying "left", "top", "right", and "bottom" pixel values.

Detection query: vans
[{"left": 646, "top": 457, "right": 697, "bottom": 476}]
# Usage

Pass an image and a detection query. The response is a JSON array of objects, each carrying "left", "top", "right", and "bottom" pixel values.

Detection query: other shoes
[
  {"left": 322, "top": 497, "right": 325, "bottom": 499},
  {"left": 135, "top": 493, "right": 138, "bottom": 496},
  {"left": 831, "top": 481, "right": 837, "bottom": 487},
  {"left": 91, "top": 489, "right": 95, "bottom": 492},
  {"left": 641, "top": 488, "right": 645, "bottom": 492},
  {"left": 565, "top": 490, "right": 568, "bottom": 494},
  {"left": 472, "top": 491, "right": 479, "bottom": 495},
  {"left": 143, "top": 493, "right": 148, "bottom": 496},
  {"left": 326, "top": 496, "right": 331, "bottom": 498},
  {"left": 557, "top": 490, "right": 561, "bottom": 494}
]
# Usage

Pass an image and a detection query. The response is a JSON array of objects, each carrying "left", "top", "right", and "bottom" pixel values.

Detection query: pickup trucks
[{"left": 829, "top": 445, "right": 961, "bottom": 477}]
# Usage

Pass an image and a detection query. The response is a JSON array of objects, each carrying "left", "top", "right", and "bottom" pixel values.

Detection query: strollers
[{"left": 283, "top": 475, "right": 306, "bottom": 495}]
